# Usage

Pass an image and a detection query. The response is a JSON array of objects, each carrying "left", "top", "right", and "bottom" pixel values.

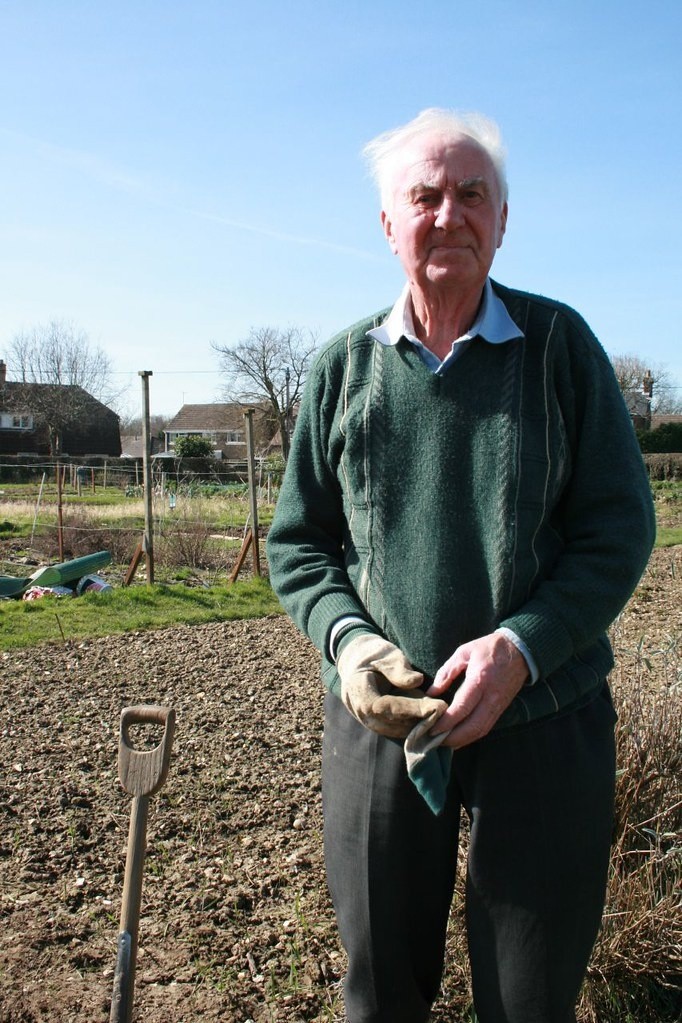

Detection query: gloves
[{"left": 332, "top": 623, "right": 452, "bottom": 815}]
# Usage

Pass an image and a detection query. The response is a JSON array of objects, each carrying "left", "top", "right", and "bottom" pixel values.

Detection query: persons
[{"left": 265, "top": 106, "right": 657, "bottom": 1023}]
